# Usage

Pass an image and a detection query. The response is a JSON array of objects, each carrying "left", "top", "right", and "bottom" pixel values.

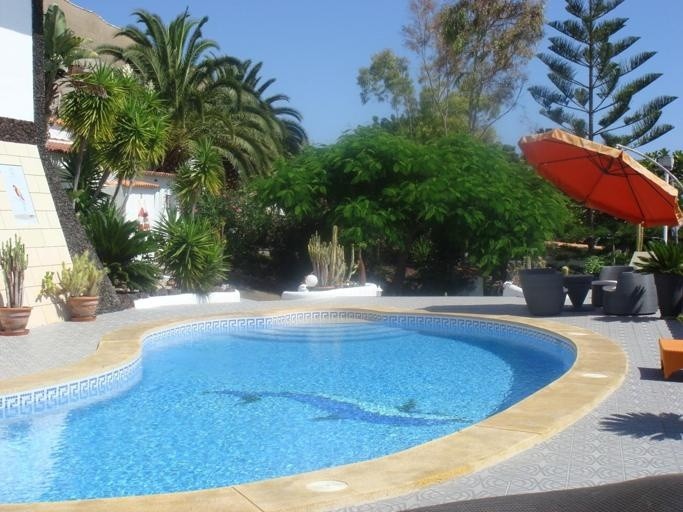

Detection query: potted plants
[
  {"left": 305, "top": 230, "right": 348, "bottom": 291},
  {"left": 632, "top": 240, "right": 682, "bottom": 318},
  {"left": 0, "top": 233, "right": 110, "bottom": 336}
]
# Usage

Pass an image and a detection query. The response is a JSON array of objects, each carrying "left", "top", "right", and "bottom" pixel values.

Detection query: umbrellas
[{"left": 518, "top": 127, "right": 683, "bottom": 245}]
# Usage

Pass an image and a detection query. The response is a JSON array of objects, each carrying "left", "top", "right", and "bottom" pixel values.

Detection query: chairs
[{"left": 518, "top": 266, "right": 659, "bottom": 316}]
